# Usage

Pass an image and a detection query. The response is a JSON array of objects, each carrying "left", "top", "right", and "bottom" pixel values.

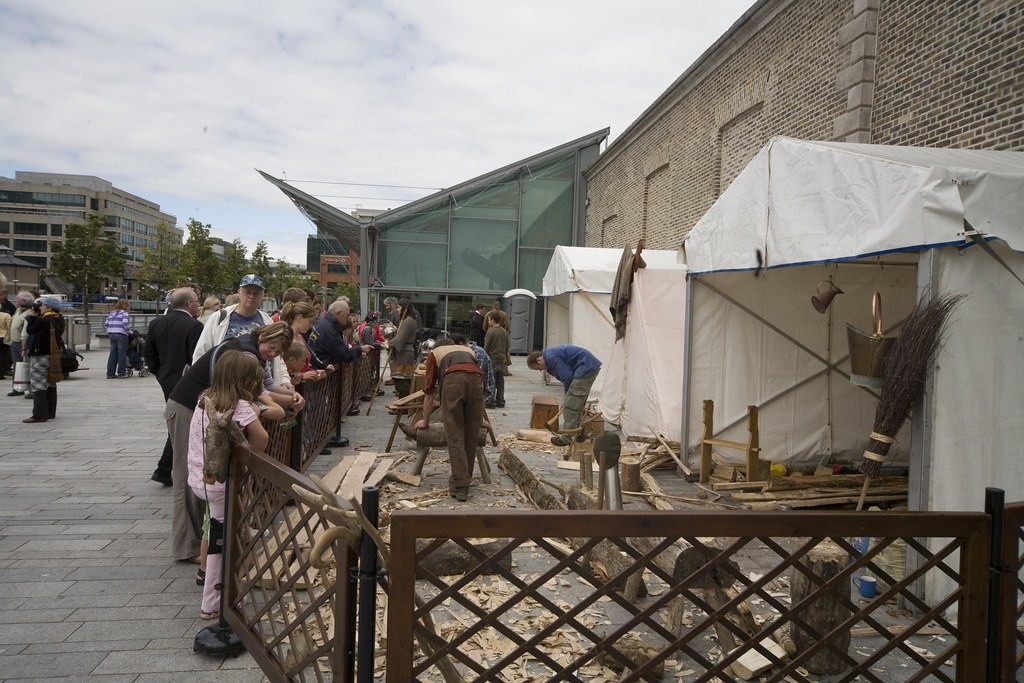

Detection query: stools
[
  {"left": 612, "top": 537, "right": 740, "bottom": 658},
  {"left": 411, "top": 422, "right": 492, "bottom": 484}
]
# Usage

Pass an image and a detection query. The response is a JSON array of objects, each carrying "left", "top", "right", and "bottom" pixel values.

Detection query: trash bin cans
[{"left": 62, "top": 316, "right": 91, "bottom": 350}]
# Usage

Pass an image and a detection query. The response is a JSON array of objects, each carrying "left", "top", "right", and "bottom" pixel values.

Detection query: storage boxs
[{"left": 530, "top": 397, "right": 560, "bottom": 430}]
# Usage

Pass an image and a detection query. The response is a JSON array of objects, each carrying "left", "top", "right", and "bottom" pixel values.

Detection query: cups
[{"left": 852, "top": 576, "right": 876, "bottom": 597}]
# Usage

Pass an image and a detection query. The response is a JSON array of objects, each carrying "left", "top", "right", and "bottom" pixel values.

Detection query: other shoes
[
  {"left": 118, "top": 373, "right": 129, "bottom": 379},
  {"left": 551, "top": 436, "right": 574, "bottom": 446},
  {"left": 503, "top": 372, "right": 513, "bottom": 376},
  {"left": 23, "top": 417, "right": 46, "bottom": 423},
  {"left": 398, "top": 421, "right": 418, "bottom": 442},
  {"left": 7, "top": 389, "right": 24, "bottom": 396},
  {"left": 239, "top": 527, "right": 270, "bottom": 543},
  {"left": 200, "top": 594, "right": 248, "bottom": 620},
  {"left": 6, "top": 371, "right": 13, "bottom": 376},
  {"left": 384, "top": 378, "right": 395, "bottom": 385},
  {"left": 449, "top": 486, "right": 470, "bottom": 501},
  {"left": 25, "top": 392, "right": 35, "bottom": 399},
  {"left": 189, "top": 554, "right": 202, "bottom": 563},
  {"left": 151, "top": 468, "right": 173, "bottom": 487},
  {"left": 107, "top": 375, "right": 118, "bottom": 379},
  {"left": 495, "top": 401, "right": 505, "bottom": 408}
]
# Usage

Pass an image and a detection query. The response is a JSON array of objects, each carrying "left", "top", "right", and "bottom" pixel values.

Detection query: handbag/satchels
[
  {"left": 60, "top": 347, "right": 84, "bottom": 379},
  {"left": 47, "top": 322, "right": 63, "bottom": 384},
  {"left": 13, "top": 356, "right": 31, "bottom": 393}
]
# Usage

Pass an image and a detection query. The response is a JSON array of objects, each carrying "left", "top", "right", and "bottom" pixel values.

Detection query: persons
[{"left": 0, "top": 274, "right": 602, "bottom": 620}]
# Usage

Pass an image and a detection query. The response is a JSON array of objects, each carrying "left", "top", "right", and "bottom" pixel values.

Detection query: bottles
[{"left": 850, "top": 538, "right": 861, "bottom": 562}]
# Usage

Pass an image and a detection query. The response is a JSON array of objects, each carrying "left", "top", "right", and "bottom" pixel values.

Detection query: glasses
[{"left": 266, "top": 341, "right": 284, "bottom": 357}]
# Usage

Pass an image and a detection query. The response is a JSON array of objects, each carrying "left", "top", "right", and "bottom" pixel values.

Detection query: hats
[
  {"left": 40, "top": 298, "right": 61, "bottom": 311},
  {"left": 239, "top": 274, "right": 267, "bottom": 290}
]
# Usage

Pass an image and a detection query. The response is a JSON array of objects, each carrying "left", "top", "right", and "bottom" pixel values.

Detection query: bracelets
[{"left": 22, "top": 348, "right": 27, "bottom": 351}]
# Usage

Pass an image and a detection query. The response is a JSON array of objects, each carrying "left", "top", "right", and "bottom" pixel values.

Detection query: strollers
[{"left": 126, "top": 329, "right": 152, "bottom": 377}]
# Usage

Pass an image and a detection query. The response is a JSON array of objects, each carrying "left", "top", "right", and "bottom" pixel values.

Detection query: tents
[{"left": 542, "top": 137, "right": 1024, "bottom": 626}]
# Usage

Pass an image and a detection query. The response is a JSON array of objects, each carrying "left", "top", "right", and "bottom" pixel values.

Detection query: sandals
[{"left": 196, "top": 568, "right": 205, "bottom": 586}]
